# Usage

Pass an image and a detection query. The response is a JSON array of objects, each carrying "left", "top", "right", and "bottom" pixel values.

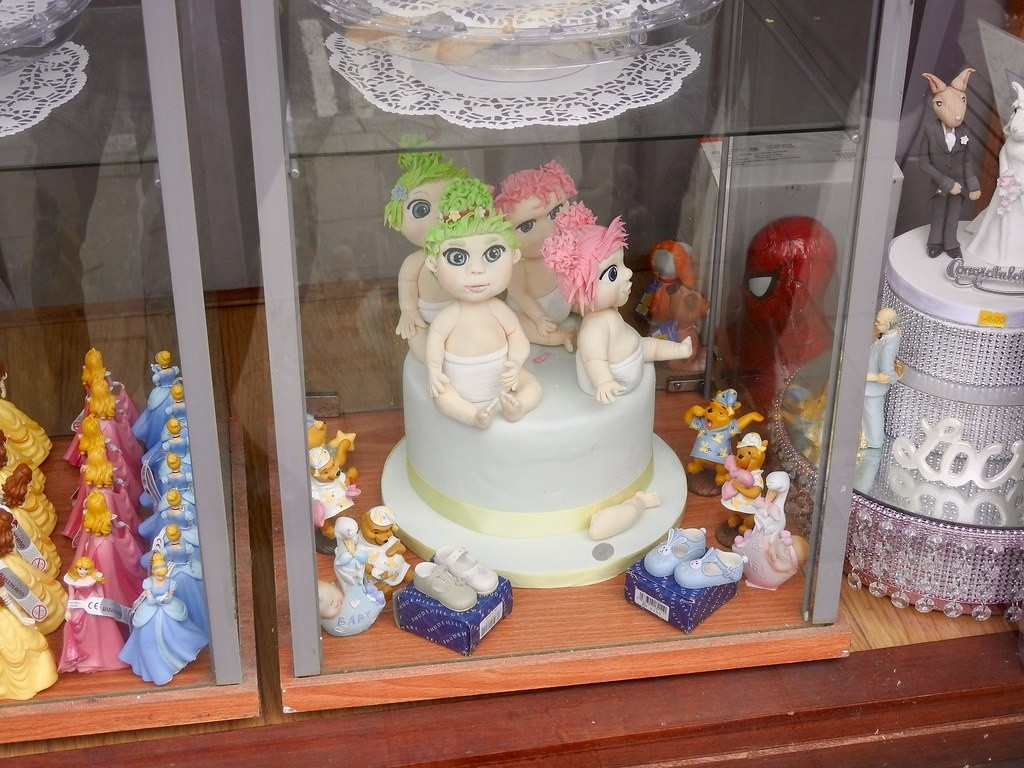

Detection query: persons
[
  {"left": 499, "top": 165, "right": 594, "bottom": 354},
  {"left": 860, "top": 308, "right": 903, "bottom": 449},
  {"left": 0, "top": 347, "right": 205, "bottom": 701},
  {"left": 635, "top": 236, "right": 710, "bottom": 371},
  {"left": 415, "top": 176, "right": 543, "bottom": 428},
  {"left": 918, "top": 68, "right": 1024, "bottom": 284},
  {"left": 542, "top": 198, "right": 695, "bottom": 404},
  {"left": 382, "top": 130, "right": 495, "bottom": 361},
  {"left": 723, "top": 213, "right": 839, "bottom": 412}
]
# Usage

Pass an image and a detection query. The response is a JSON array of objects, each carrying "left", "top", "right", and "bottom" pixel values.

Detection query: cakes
[
  {"left": 383, "top": 131, "right": 695, "bottom": 541},
  {"left": 878, "top": 67, "right": 1024, "bottom": 462}
]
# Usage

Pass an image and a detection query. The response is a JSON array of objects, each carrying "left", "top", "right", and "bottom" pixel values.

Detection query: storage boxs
[
  {"left": 661, "top": 134, "right": 907, "bottom": 331},
  {"left": 391, "top": 554, "right": 513, "bottom": 656},
  {"left": 625, "top": 547, "right": 740, "bottom": 634}
]
